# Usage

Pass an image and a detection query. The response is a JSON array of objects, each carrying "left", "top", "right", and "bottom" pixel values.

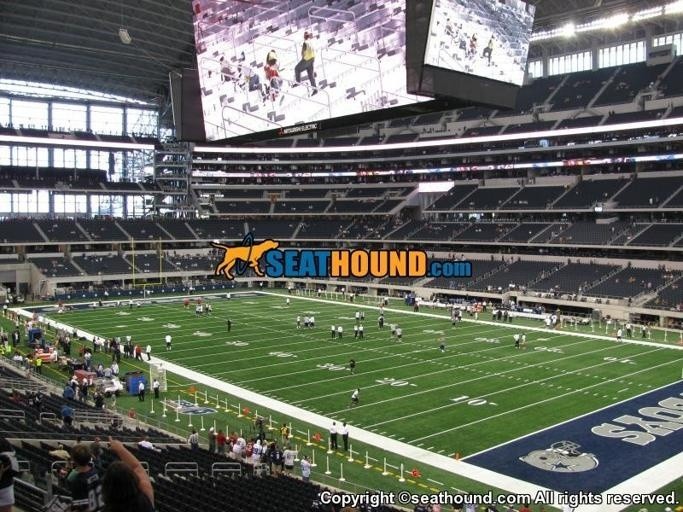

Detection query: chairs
[
  {"left": 0, "top": 363, "right": 406, "bottom": 512},
  {"left": 391, "top": 57, "right": 682, "bottom": 123},
  {"left": 0, "top": 122, "right": 683, "bottom": 318}
]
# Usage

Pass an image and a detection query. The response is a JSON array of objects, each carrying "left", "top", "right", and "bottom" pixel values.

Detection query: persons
[
  {"left": 195, "top": 2, "right": 319, "bottom": 99},
  {"left": 443, "top": 3, "right": 498, "bottom": 68}
]
